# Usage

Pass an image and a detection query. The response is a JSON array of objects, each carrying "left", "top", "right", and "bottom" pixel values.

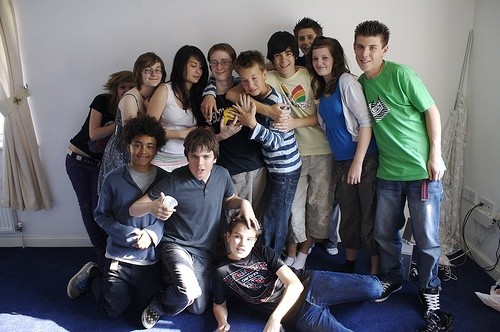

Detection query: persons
[
  {"left": 67, "top": 111, "right": 172, "bottom": 319},
  {"left": 129, "top": 126, "right": 260, "bottom": 328},
  {"left": 65, "top": 17, "right": 446, "bottom": 319},
  {"left": 210, "top": 211, "right": 382, "bottom": 332}
]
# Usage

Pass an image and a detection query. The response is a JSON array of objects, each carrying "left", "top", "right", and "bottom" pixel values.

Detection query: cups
[
  {"left": 163, "top": 196, "right": 178, "bottom": 209},
  {"left": 222, "top": 108, "right": 240, "bottom": 126}
]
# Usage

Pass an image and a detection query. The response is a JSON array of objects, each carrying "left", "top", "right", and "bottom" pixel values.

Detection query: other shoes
[
  {"left": 440, "top": 265, "right": 451, "bottom": 281},
  {"left": 140, "top": 302, "right": 161, "bottom": 328}
]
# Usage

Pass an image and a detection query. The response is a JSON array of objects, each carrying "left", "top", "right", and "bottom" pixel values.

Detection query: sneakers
[
  {"left": 68, "top": 261, "right": 99, "bottom": 300},
  {"left": 373, "top": 281, "right": 403, "bottom": 302},
  {"left": 418, "top": 286, "right": 440, "bottom": 318}
]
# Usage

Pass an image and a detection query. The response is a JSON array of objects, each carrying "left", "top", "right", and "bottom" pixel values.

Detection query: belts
[{"left": 67, "top": 148, "right": 101, "bottom": 167}]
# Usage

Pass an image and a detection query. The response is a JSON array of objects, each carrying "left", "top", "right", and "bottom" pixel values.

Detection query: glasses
[
  {"left": 209, "top": 59, "right": 233, "bottom": 67},
  {"left": 142, "top": 69, "right": 164, "bottom": 74}
]
